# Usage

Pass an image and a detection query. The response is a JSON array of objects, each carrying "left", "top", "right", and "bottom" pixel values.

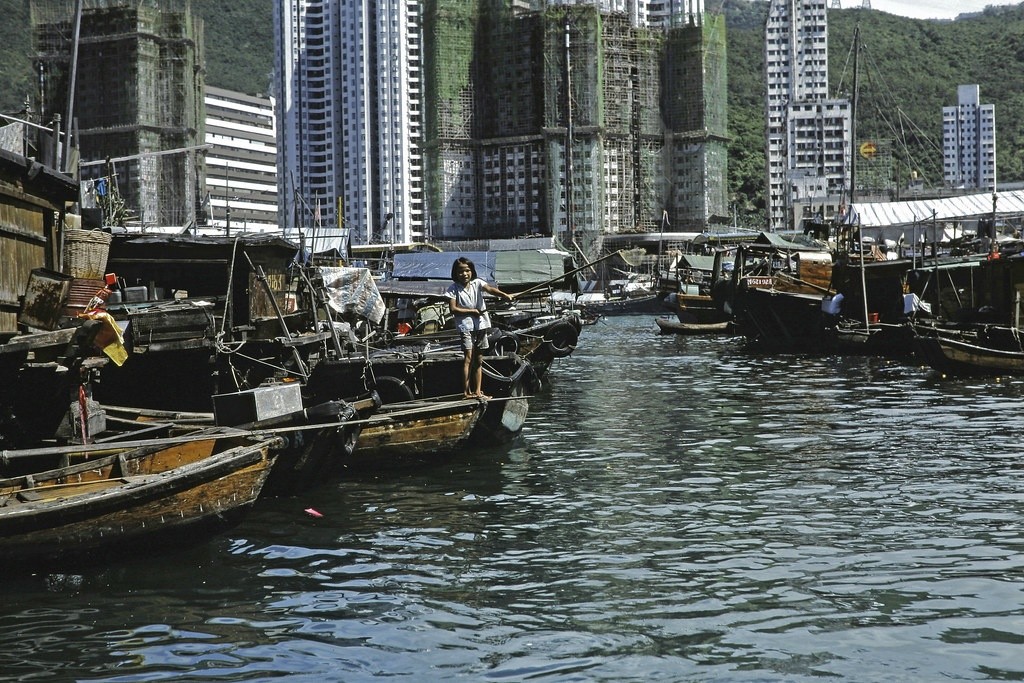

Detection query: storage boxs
[{"left": 16, "top": 266, "right": 74, "bottom": 330}]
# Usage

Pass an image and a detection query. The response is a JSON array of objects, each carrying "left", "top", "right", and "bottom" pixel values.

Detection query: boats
[
  {"left": 557, "top": 28, "right": 1024, "bottom": 380},
  {"left": 287, "top": 281, "right": 588, "bottom": 429},
  {"left": 74, "top": 386, "right": 492, "bottom": 465},
  {"left": 0, "top": 425, "right": 289, "bottom": 570}
]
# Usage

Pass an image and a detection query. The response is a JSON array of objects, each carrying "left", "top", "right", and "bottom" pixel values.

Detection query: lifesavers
[
  {"left": 491, "top": 332, "right": 520, "bottom": 356},
  {"left": 544, "top": 322, "right": 578, "bottom": 357}
]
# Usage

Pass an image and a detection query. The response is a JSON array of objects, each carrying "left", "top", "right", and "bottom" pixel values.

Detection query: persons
[{"left": 443, "top": 257, "right": 515, "bottom": 399}]
